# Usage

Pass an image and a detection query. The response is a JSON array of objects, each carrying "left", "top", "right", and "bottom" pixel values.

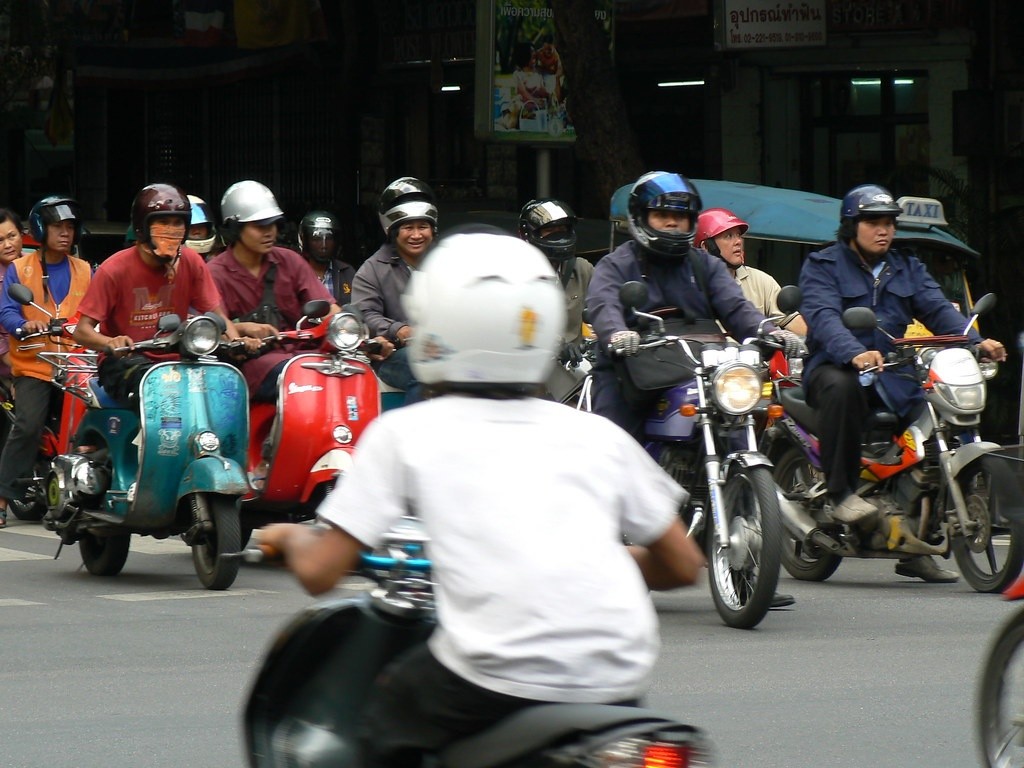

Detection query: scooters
[
  {"left": 214, "top": 514, "right": 722, "bottom": 767},
  {"left": 0, "top": 275, "right": 411, "bottom": 592}
]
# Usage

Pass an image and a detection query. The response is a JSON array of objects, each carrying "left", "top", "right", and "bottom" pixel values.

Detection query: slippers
[{"left": 0, "top": 507, "right": 7, "bottom": 528}]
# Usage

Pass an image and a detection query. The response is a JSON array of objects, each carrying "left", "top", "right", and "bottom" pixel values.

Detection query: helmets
[
  {"left": 379, "top": 177, "right": 439, "bottom": 234},
  {"left": 694, "top": 208, "right": 749, "bottom": 248},
  {"left": 519, "top": 198, "right": 577, "bottom": 261},
  {"left": 183, "top": 195, "right": 216, "bottom": 253},
  {"left": 298, "top": 211, "right": 343, "bottom": 263},
  {"left": 221, "top": 179, "right": 284, "bottom": 225},
  {"left": 840, "top": 184, "right": 904, "bottom": 218},
  {"left": 398, "top": 225, "right": 567, "bottom": 388},
  {"left": 627, "top": 171, "right": 703, "bottom": 258},
  {"left": 29, "top": 196, "right": 82, "bottom": 246},
  {"left": 130, "top": 183, "right": 192, "bottom": 245}
]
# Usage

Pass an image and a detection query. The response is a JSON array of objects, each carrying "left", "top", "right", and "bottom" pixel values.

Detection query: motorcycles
[{"left": 543, "top": 179, "right": 1024, "bottom": 631}]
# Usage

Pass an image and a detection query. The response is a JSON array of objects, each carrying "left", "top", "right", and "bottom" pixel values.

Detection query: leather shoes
[
  {"left": 773, "top": 594, "right": 795, "bottom": 607},
  {"left": 831, "top": 494, "right": 878, "bottom": 524},
  {"left": 894, "top": 554, "right": 959, "bottom": 582}
]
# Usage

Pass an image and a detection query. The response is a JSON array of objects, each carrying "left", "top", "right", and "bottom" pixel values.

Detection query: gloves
[
  {"left": 609, "top": 331, "right": 639, "bottom": 357},
  {"left": 769, "top": 330, "right": 809, "bottom": 357},
  {"left": 559, "top": 342, "right": 583, "bottom": 367}
]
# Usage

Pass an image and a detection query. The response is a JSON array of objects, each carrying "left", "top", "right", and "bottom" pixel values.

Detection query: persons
[
  {"left": 518, "top": 197, "right": 594, "bottom": 413},
  {"left": 499, "top": 34, "right": 566, "bottom": 128},
  {"left": 73, "top": 183, "right": 261, "bottom": 501},
  {"left": 0, "top": 197, "right": 355, "bottom": 528},
  {"left": 205, "top": 181, "right": 394, "bottom": 489},
  {"left": 799, "top": 184, "right": 1005, "bottom": 583},
  {"left": 253, "top": 223, "right": 706, "bottom": 768},
  {"left": 351, "top": 175, "right": 438, "bottom": 402},
  {"left": 586, "top": 169, "right": 808, "bottom": 606},
  {"left": 692, "top": 208, "right": 807, "bottom": 337}
]
